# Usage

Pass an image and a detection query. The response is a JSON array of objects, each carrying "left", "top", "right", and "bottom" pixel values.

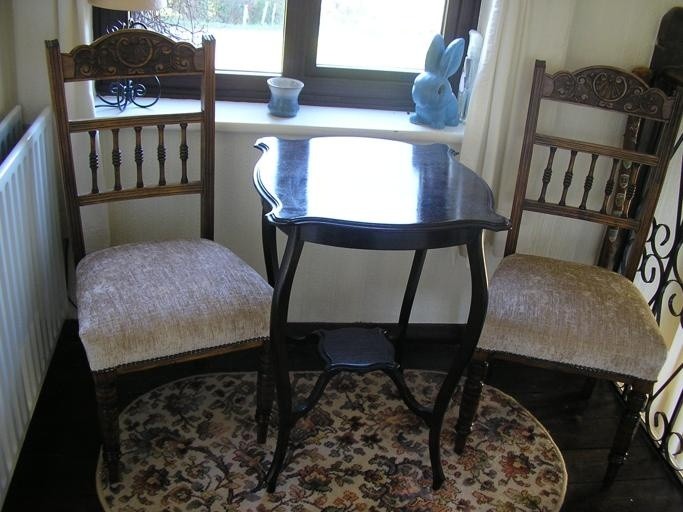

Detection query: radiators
[
  {"left": 0, "top": 106, "right": 72, "bottom": 512},
  {"left": 0, "top": 103, "right": 25, "bottom": 163}
]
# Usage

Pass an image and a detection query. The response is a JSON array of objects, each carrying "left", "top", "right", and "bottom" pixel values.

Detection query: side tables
[{"left": 252, "top": 134, "right": 514, "bottom": 495}]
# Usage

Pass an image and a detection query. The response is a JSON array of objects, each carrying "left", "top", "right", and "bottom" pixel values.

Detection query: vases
[{"left": 265, "top": 76, "right": 307, "bottom": 120}]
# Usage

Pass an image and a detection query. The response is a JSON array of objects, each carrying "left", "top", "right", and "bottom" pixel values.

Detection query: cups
[{"left": 266, "top": 78, "right": 304, "bottom": 119}]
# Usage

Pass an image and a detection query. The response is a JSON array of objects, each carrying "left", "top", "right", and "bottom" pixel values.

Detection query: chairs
[
  {"left": 453, "top": 57, "right": 682, "bottom": 491},
  {"left": 44, "top": 28, "right": 279, "bottom": 486}
]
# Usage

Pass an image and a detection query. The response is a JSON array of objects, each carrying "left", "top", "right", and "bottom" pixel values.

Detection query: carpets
[{"left": 94, "top": 364, "right": 570, "bottom": 511}]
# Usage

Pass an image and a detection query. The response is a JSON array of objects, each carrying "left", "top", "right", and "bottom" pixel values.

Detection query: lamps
[{"left": 87, "top": 0, "right": 163, "bottom": 112}]
